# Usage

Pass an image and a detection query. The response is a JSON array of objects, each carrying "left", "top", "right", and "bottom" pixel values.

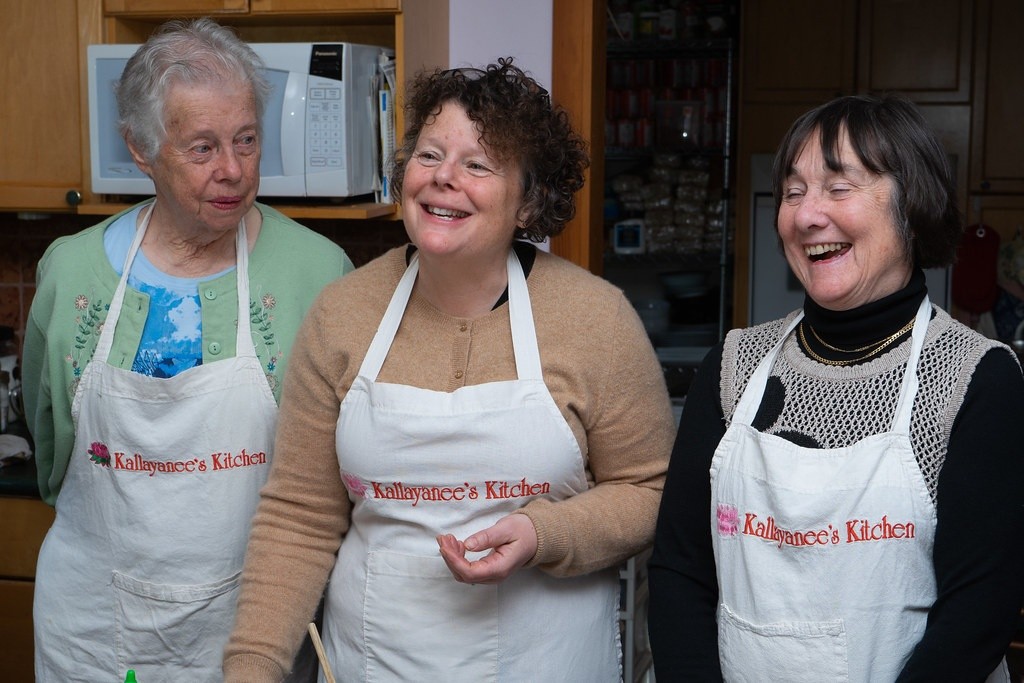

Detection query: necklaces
[{"left": 797, "top": 316, "right": 915, "bottom": 365}]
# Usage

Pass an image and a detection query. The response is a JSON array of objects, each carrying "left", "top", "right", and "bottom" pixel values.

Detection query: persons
[
  {"left": 14, "top": 18, "right": 353, "bottom": 681},
  {"left": 651, "top": 96, "right": 1023, "bottom": 683},
  {"left": 223, "top": 60, "right": 668, "bottom": 683}
]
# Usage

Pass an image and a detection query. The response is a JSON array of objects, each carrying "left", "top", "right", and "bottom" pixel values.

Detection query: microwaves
[{"left": 87, "top": 43, "right": 393, "bottom": 204}]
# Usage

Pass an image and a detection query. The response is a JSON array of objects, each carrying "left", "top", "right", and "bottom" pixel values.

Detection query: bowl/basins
[{"left": 656, "top": 269, "right": 711, "bottom": 298}]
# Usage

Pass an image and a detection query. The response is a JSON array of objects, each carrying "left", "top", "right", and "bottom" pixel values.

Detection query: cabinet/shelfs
[{"left": 734, "top": 0, "right": 1023, "bottom": 329}]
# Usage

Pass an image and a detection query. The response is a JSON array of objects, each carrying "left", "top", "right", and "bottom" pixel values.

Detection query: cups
[{"left": 634, "top": 297, "right": 670, "bottom": 334}]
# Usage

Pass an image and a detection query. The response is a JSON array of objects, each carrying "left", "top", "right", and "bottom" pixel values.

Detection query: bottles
[
  {"left": 614, "top": 209, "right": 645, "bottom": 256},
  {"left": 0, "top": 370, "right": 10, "bottom": 433},
  {"left": 606, "top": 1, "right": 735, "bottom": 40},
  {"left": 0, "top": 325, "right": 20, "bottom": 423}
]
